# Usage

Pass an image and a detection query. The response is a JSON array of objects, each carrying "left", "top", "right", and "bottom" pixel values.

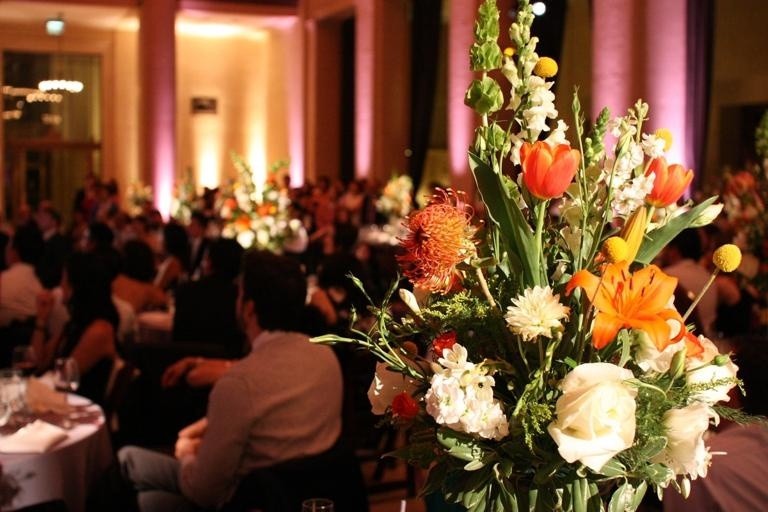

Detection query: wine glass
[{"left": 54, "top": 357, "right": 80, "bottom": 429}]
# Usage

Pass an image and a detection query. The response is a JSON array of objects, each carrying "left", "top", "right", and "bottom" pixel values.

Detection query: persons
[
  {"left": 654, "top": 150, "right": 768, "bottom": 434},
  {"left": 0, "top": 164, "right": 445, "bottom": 512}
]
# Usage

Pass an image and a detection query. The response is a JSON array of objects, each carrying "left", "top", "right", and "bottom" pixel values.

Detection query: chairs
[
  {"left": 225, "top": 451, "right": 373, "bottom": 510},
  {"left": 336, "top": 355, "right": 417, "bottom": 501}
]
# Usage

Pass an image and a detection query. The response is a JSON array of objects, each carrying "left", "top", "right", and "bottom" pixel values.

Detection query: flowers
[
  {"left": 211, "top": 148, "right": 309, "bottom": 256},
  {"left": 311, "top": 0, "right": 750, "bottom": 512}
]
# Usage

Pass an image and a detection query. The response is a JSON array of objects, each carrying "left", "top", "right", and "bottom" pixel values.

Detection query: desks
[{"left": 0, "top": 379, "right": 107, "bottom": 511}]
